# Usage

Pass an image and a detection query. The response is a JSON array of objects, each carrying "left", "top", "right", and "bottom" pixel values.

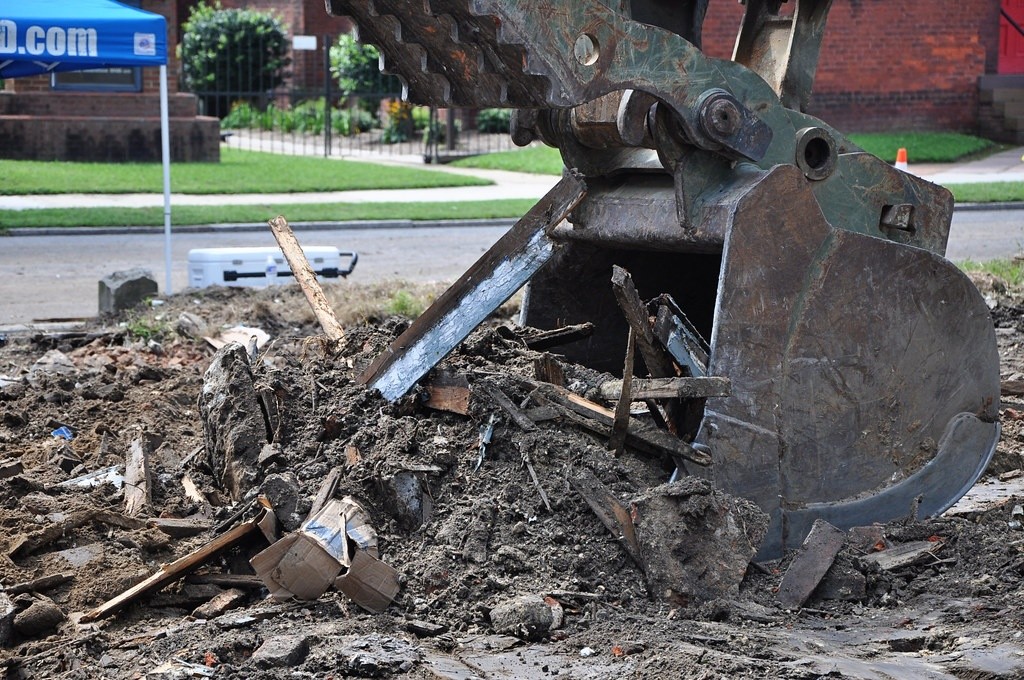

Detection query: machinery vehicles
[{"left": 316, "top": 0, "right": 1005, "bottom": 561}]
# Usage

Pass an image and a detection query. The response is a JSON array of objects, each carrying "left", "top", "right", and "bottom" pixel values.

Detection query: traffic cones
[{"left": 894, "top": 146, "right": 908, "bottom": 173}]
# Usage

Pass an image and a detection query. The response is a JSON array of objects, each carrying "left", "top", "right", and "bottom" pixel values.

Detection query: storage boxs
[{"left": 186, "top": 245, "right": 358, "bottom": 290}]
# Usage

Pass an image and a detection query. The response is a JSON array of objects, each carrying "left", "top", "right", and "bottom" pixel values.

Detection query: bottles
[{"left": 895, "top": 148, "right": 908, "bottom": 172}]
